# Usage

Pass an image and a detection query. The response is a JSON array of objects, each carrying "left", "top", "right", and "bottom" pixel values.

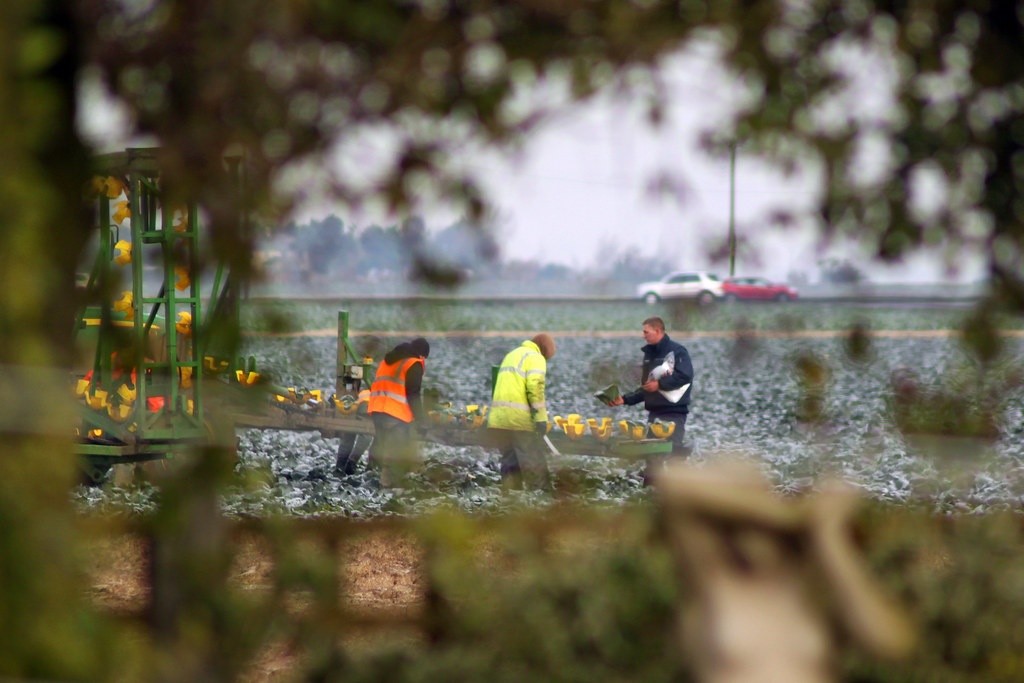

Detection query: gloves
[{"left": 536, "top": 421, "right": 546, "bottom": 435}]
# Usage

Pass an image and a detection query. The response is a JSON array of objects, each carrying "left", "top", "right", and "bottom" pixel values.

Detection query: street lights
[{"left": 713, "top": 119, "right": 748, "bottom": 276}]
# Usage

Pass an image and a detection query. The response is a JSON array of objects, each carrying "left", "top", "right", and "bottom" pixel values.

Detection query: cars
[{"left": 721, "top": 275, "right": 797, "bottom": 304}]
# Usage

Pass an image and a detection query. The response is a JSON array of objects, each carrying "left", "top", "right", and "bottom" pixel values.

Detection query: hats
[
  {"left": 531, "top": 333, "right": 555, "bottom": 359},
  {"left": 411, "top": 338, "right": 430, "bottom": 358}
]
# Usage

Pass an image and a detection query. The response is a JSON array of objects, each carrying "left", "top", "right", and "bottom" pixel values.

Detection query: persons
[
  {"left": 487, "top": 335, "right": 554, "bottom": 496},
  {"left": 333, "top": 336, "right": 430, "bottom": 485},
  {"left": 610, "top": 317, "right": 693, "bottom": 487}
]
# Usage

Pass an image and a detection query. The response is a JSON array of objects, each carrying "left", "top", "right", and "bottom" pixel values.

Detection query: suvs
[{"left": 635, "top": 268, "right": 724, "bottom": 308}]
